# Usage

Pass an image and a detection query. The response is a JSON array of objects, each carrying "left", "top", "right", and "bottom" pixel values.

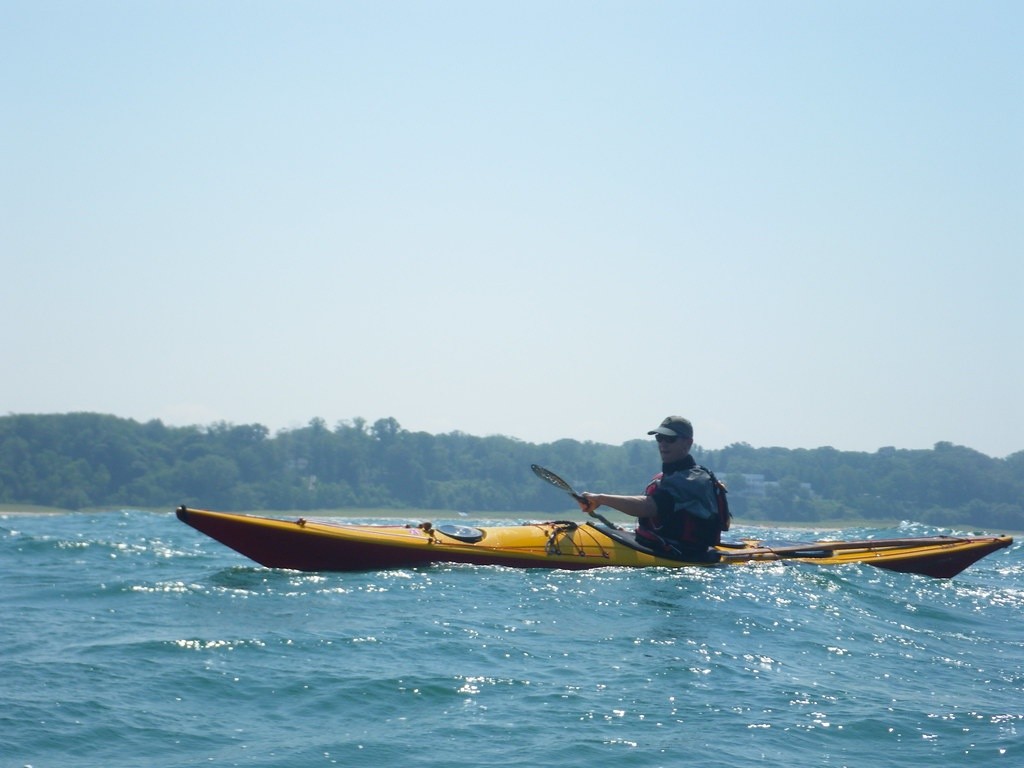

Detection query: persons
[{"left": 577, "top": 415, "right": 720, "bottom": 557}]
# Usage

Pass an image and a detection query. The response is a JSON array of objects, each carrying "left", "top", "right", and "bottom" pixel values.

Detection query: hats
[{"left": 648, "top": 415, "right": 693, "bottom": 441}]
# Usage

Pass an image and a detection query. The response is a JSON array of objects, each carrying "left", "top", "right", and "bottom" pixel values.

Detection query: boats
[{"left": 174, "top": 505, "right": 1014, "bottom": 581}]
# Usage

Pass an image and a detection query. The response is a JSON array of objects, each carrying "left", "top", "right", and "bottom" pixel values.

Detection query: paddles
[{"left": 527, "top": 459, "right": 627, "bottom": 537}]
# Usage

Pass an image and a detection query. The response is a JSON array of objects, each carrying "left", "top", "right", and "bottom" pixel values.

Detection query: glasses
[{"left": 655, "top": 435, "right": 689, "bottom": 444}]
